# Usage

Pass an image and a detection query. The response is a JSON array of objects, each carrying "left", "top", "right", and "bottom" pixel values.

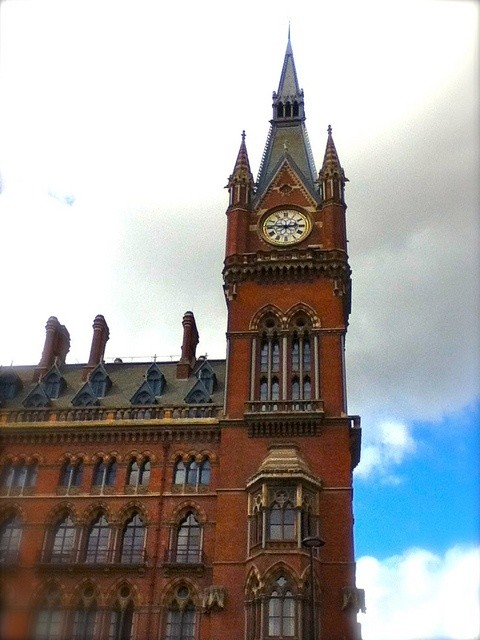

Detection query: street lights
[{"left": 301, "top": 536, "right": 326, "bottom": 640}]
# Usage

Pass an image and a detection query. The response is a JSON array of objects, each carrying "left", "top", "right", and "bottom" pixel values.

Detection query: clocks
[{"left": 263, "top": 208, "right": 310, "bottom": 246}]
